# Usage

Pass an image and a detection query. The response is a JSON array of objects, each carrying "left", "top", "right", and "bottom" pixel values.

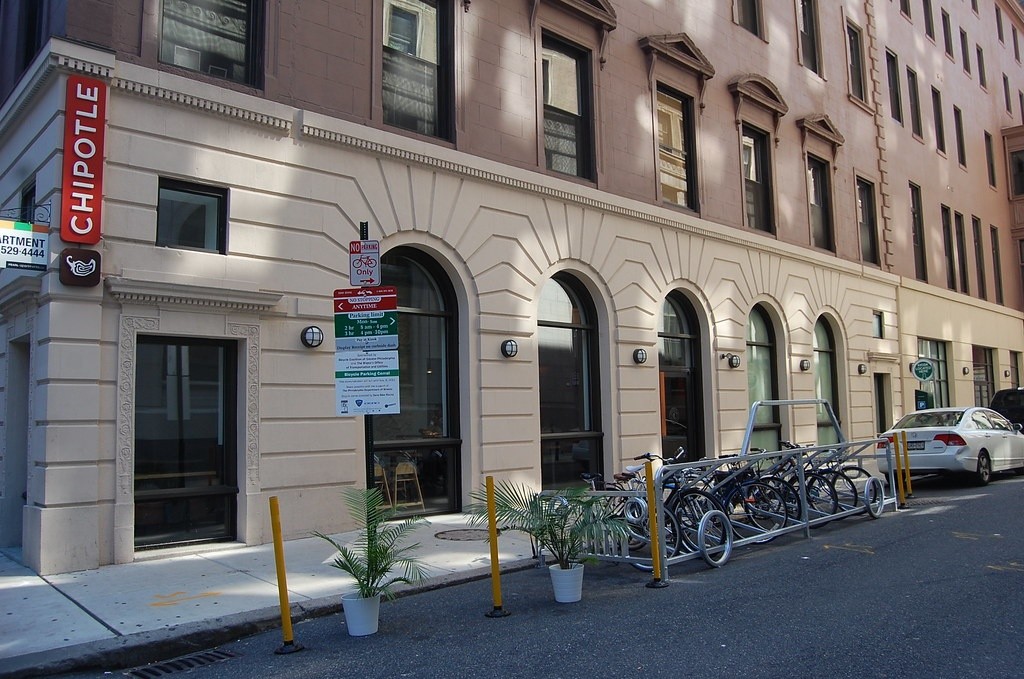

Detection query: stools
[
  {"left": 374, "top": 463, "right": 395, "bottom": 516},
  {"left": 390, "top": 460, "right": 425, "bottom": 516}
]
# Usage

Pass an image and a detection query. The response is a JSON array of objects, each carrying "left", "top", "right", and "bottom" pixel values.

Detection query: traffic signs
[
  {"left": 334, "top": 313, "right": 399, "bottom": 352},
  {"left": 333, "top": 288, "right": 398, "bottom": 312}
]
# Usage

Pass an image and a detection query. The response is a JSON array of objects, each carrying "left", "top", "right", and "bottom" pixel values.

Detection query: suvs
[{"left": 991, "top": 387, "right": 1024, "bottom": 436}]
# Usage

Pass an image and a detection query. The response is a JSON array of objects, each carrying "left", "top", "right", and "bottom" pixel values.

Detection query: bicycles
[{"left": 533, "top": 439, "right": 879, "bottom": 573}]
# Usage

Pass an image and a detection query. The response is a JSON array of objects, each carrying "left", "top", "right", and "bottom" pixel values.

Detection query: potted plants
[
  {"left": 462, "top": 478, "right": 649, "bottom": 603},
  {"left": 306, "top": 486, "right": 432, "bottom": 636}
]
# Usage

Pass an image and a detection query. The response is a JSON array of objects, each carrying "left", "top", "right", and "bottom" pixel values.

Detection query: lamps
[
  {"left": 301, "top": 326, "right": 324, "bottom": 348},
  {"left": 501, "top": 339, "right": 518, "bottom": 358},
  {"left": 729, "top": 354, "right": 740, "bottom": 368},
  {"left": 800, "top": 360, "right": 810, "bottom": 371},
  {"left": 963, "top": 367, "right": 969, "bottom": 375},
  {"left": 1005, "top": 370, "right": 1010, "bottom": 377},
  {"left": 633, "top": 349, "right": 647, "bottom": 364},
  {"left": 910, "top": 363, "right": 915, "bottom": 372},
  {"left": 858, "top": 364, "right": 867, "bottom": 374}
]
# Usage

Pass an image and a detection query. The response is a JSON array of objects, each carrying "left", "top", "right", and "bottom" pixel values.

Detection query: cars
[{"left": 877, "top": 407, "right": 1024, "bottom": 486}]
